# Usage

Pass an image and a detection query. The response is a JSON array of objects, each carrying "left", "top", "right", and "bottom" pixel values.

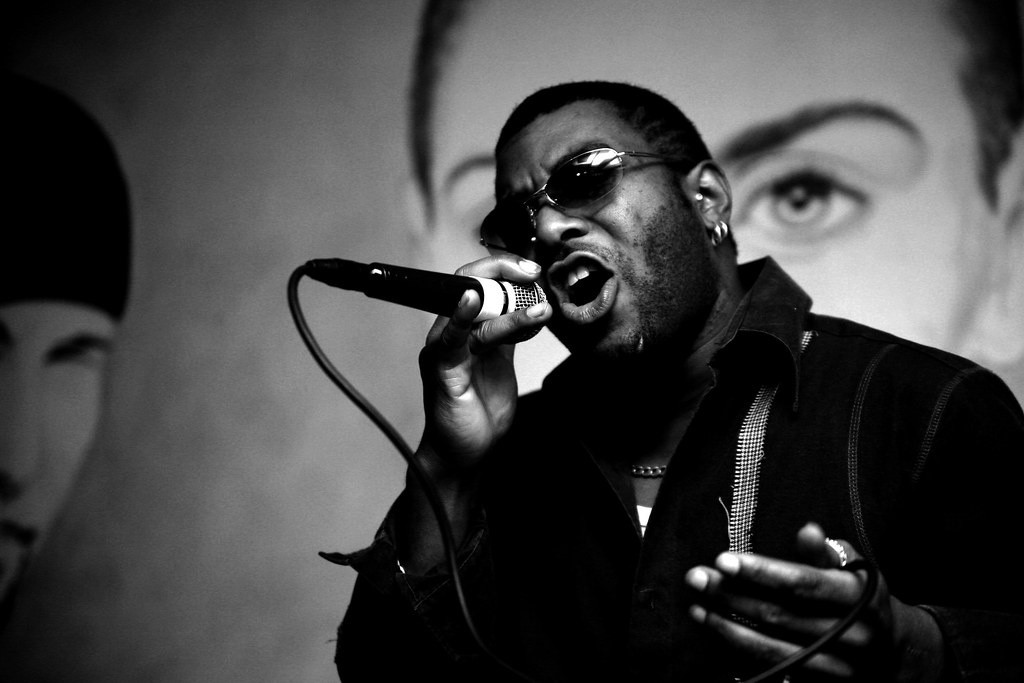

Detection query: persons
[{"left": 318, "top": 80, "right": 1024, "bottom": 683}]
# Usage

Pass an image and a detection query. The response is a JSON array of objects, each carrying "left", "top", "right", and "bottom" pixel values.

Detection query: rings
[{"left": 824, "top": 537, "right": 847, "bottom": 567}]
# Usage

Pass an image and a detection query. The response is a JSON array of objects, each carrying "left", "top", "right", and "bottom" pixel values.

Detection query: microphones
[{"left": 304, "top": 258, "right": 547, "bottom": 343}]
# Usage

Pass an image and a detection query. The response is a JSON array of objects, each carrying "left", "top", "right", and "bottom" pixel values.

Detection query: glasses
[{"left": 480, "top": 148, "right": 693, "bottom": 260}]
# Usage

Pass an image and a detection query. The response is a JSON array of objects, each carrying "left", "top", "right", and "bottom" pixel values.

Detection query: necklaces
[{"left": 630, "top": 463, "right": 667, "bottom": 478}]
